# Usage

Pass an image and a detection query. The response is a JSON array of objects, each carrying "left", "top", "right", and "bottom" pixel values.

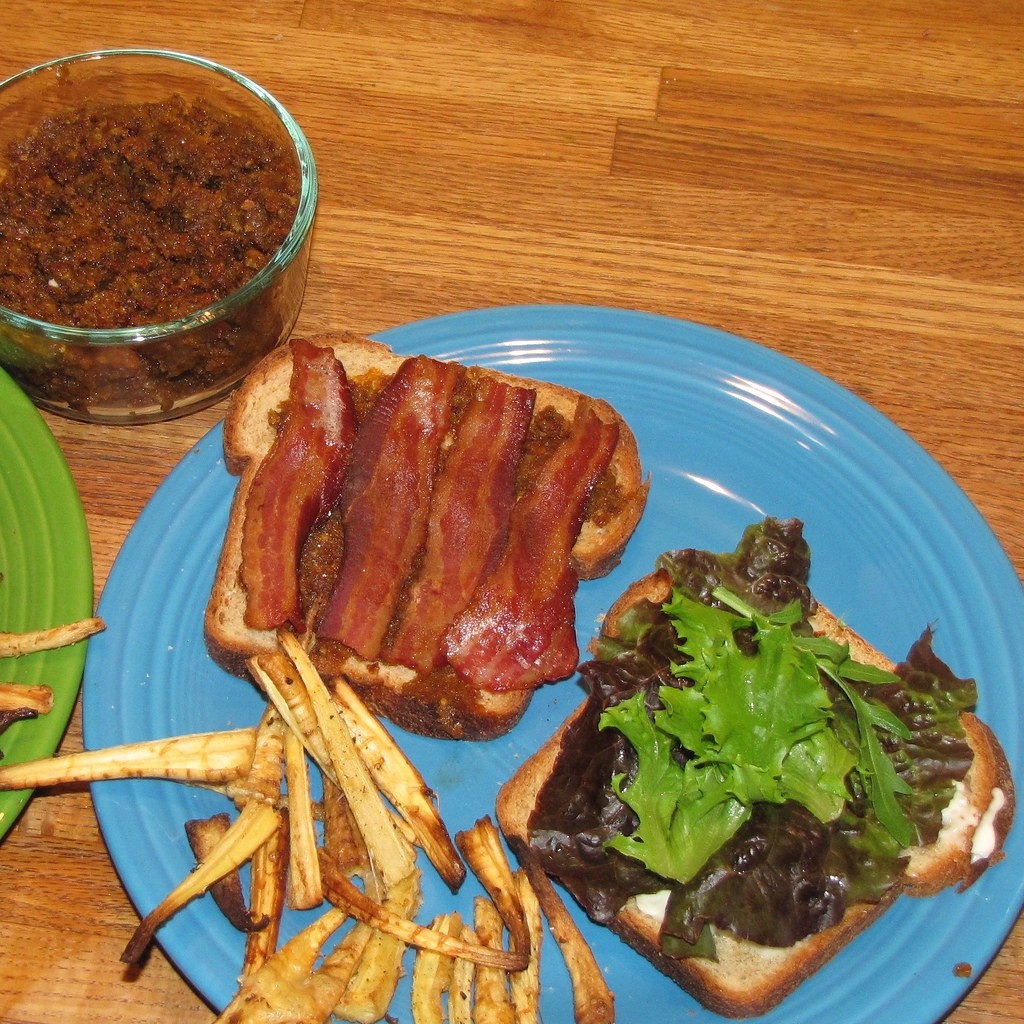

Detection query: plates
[
  {"left": 0, "top": 366, "right": 92, "bottom": 840},
  {"left": 78, "top": 302, "right": 1023, "bottom": 1024}
]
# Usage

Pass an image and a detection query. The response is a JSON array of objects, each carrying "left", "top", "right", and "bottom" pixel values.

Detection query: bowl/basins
[{"left": 0, "top": 48, "right": 318, "bottom": 425}]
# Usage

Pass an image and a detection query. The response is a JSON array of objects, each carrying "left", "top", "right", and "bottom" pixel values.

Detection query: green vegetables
[{"left": 524, "top": 515, "right": 979, "bottom": 963}]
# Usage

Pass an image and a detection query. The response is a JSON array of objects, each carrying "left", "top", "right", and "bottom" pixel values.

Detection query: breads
[
  {"left": 206, "top": 330, "right": 648, "bottom": 742},
  {"left": 495, "top": 567, "right": 1018, "bottom": 1022}
]
